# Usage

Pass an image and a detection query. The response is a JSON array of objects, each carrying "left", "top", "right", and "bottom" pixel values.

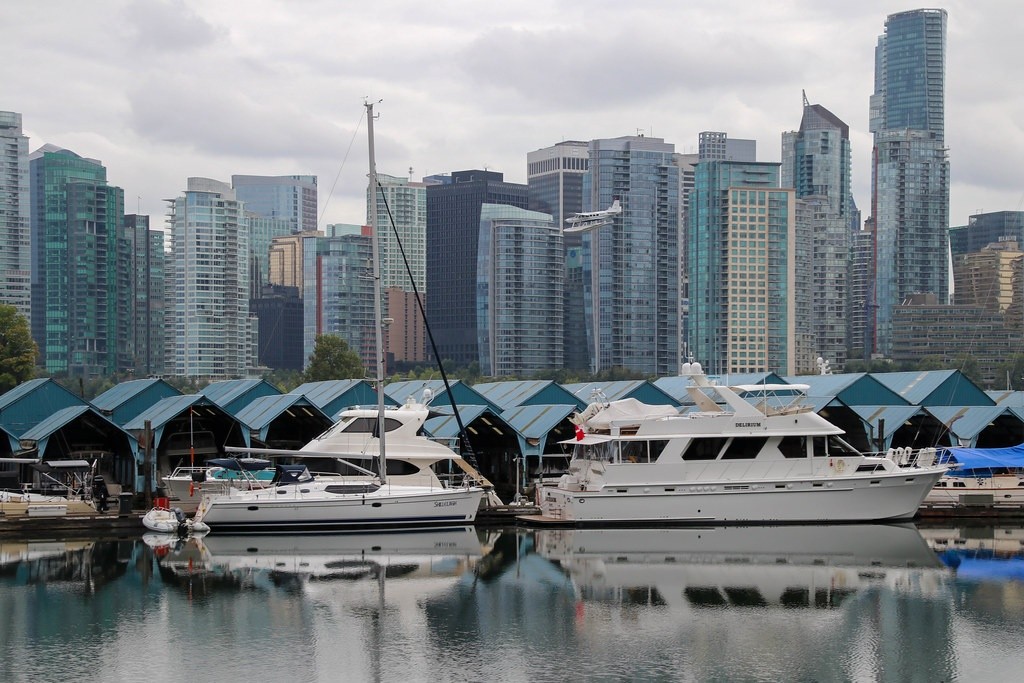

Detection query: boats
[
  {"left": 141, "top": 528, "right": 204, "bottom": 554},
  {"left": 531, "top": 525, "right": 954, "bottom": 604},
  {"left": 142, "top": 506, "right": 211, "bottom": 533},
  {"left": 508, "top": 360, "right": 954, "bottom": 526},
  {"left": 895, "top": 442, "right": 1024, "bottom": 518}
]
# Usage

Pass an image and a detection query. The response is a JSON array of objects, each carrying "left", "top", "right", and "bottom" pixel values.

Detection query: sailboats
[{"left": 156, "top": 95, "right": 492, "bottom": 536}]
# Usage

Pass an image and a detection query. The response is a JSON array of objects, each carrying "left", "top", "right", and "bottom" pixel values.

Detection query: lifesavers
[
  {"left": 835, "top": 459, "right": 846, "bottom": 473},
  {"left": 189, "top": 482, "right": 194, "bottom": 497}
]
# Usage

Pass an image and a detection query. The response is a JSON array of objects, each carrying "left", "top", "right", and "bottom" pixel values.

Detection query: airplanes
[{"left": 564, "top": 201, "right": 624, "bottom": 225}]
